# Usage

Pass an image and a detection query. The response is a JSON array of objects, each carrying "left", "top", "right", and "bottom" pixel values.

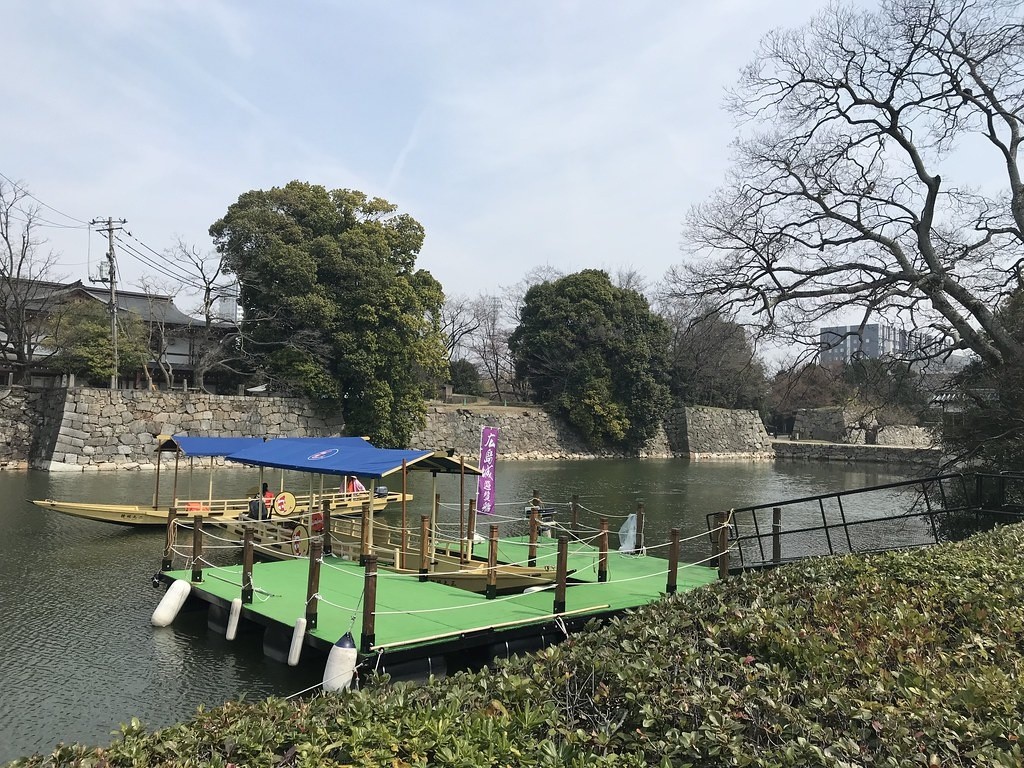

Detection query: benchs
[{"left": 168, "top": 387, "right": 200, "bottom": 394}]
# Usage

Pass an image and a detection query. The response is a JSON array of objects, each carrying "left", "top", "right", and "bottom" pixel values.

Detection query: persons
[
  {"left": 339, "top": 475, "right": 365, "bottom": 501},
  {"left": 254, "top": 483, "right": 273, "bottom": 508}
]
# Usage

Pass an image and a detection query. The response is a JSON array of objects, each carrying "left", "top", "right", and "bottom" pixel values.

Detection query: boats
[
  {"left": 23, "top": 436, "right": 414, "bottom": 529},
  {"left": 213, "top": 440, "right": 578, "bottom": 597}
]
